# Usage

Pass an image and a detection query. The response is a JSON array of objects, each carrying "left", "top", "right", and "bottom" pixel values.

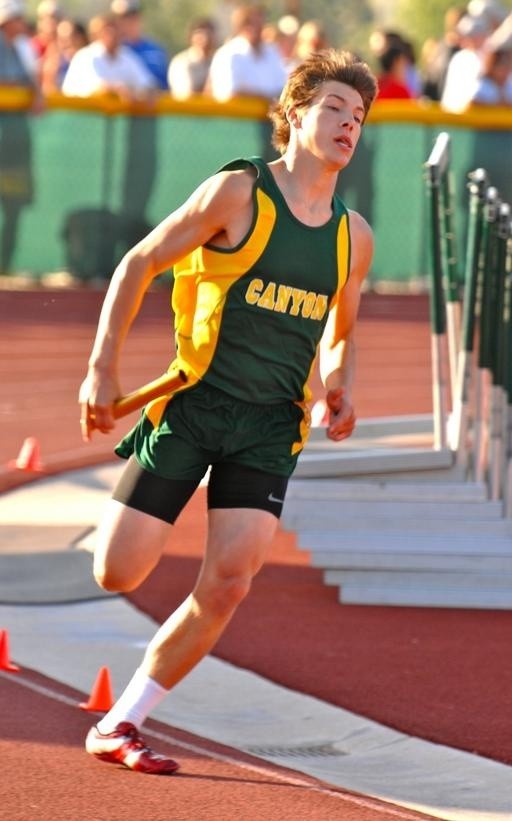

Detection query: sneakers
[{"left": 85, "top": 721, "right": 180, "bottom": 774}]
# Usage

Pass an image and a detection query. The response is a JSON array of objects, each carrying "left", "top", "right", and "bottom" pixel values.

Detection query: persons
[
  {"left": 0, "top": 0, "right": 169, "bottom": 99},
  {"left": 78, "top": 46, "right": 378, "bottom": 775},
  {"left": 166, "top": 0, "right": 325, "bottom": 101},
  {"left": 366, "top": 0, "right": 511, "bottom": 111}
]
